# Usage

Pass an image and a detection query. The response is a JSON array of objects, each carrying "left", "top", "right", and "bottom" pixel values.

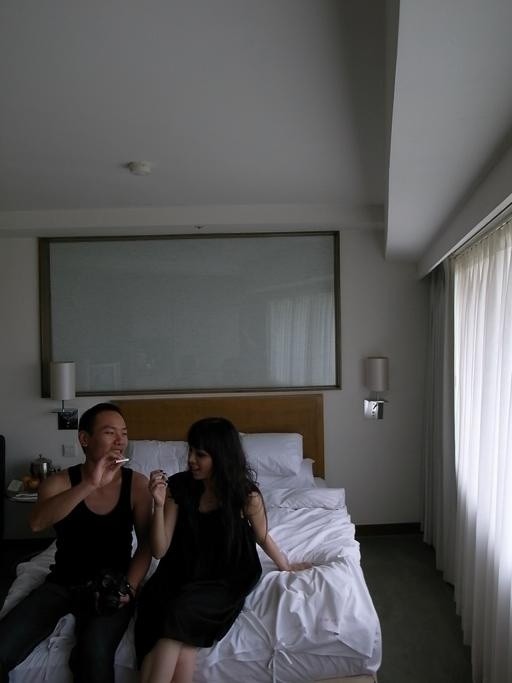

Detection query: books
[
  {"left": 12, "top": 493, "right": 38, "bottom": 500},
  {"left": 16, "top": 489, "right": 38, "bottom": 496}
]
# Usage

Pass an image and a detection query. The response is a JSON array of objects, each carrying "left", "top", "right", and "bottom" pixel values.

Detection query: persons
[
  {"left": 132, "top": 415, "right": 325, "bottom": 682},
  {"left": 1, "top": 402, "right": 154, "bottom": 682}
]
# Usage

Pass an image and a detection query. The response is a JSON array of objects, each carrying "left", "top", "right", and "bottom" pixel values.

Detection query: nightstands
[{"left": 8, "top": 489, "right": 57, "bottom": 554}]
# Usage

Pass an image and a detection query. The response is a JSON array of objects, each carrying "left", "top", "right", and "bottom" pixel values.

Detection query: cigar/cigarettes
[
  {"left": 159, "top": 471, "right": 168, "bottom": 479},
  {"left": 112, "top": 456, "right": 131, "bottom": 464}
]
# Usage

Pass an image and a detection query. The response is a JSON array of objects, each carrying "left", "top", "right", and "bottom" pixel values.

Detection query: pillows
[{"left": 119, "top": 431, "right": 315, "bottom": 493}]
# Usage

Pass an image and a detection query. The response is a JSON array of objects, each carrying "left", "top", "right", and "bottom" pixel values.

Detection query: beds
[{"left": 2, "top": 394, "right": 383, "bottom": 682}]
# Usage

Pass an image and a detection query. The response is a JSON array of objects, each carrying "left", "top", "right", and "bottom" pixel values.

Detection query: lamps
[
  {"left": 361, "top": 357, "right": 388, "bottom": 420},
  {"left": 51, "top": 363, "right": 78, "bottom": 431}
]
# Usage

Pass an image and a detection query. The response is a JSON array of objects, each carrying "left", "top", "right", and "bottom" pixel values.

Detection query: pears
[
  {"left": 21, "top": 474, "right": 32, "bottom": 488},
  {"left": 29, "top": 479, "right": 40, "bottom": 488}
]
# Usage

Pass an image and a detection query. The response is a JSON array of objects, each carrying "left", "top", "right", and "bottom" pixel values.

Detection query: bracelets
[
  {"left": 127, "top": 581, "right": 138, "bottom": 594},
  {"left": 124, "top": 581, "right": 138, "bottom": 603}
]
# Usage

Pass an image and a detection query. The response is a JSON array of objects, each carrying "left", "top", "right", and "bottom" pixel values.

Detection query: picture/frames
[{"left": 38, "top": 230, "right": 343, "bottom": 399}]
